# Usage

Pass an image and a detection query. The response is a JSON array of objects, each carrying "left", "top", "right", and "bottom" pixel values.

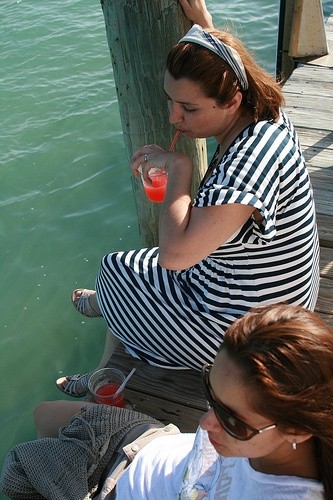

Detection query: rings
[{"left": 144, "top": 155, "right": 147, "bottom": 160}]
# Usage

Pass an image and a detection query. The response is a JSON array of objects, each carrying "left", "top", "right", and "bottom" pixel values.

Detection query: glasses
[{"left": 200, "top": 362, "right": 277, "bottom": 442}]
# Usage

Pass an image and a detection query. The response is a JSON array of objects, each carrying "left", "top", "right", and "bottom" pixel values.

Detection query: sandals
[
  {"left": 55, "top": 368, "right": 94, "bottom": 398},
  {"left": 71, "top": 288, "right": 104, "bottom": 318}
]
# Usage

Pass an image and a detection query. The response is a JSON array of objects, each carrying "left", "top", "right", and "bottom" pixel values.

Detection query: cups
[
  {"left": 137, "top": 165, "right": 168, "bottom": 204},
  {"left": 87, "top": 368, "right": 127, "bottom": 408}
]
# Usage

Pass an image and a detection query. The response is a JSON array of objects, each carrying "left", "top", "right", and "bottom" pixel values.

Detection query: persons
[
  {"left": 0, "top": 305, "right": 333, "bottom": 500},
  {"left": 55, "top": 0, "right": 319, "bottom": 398}
]
